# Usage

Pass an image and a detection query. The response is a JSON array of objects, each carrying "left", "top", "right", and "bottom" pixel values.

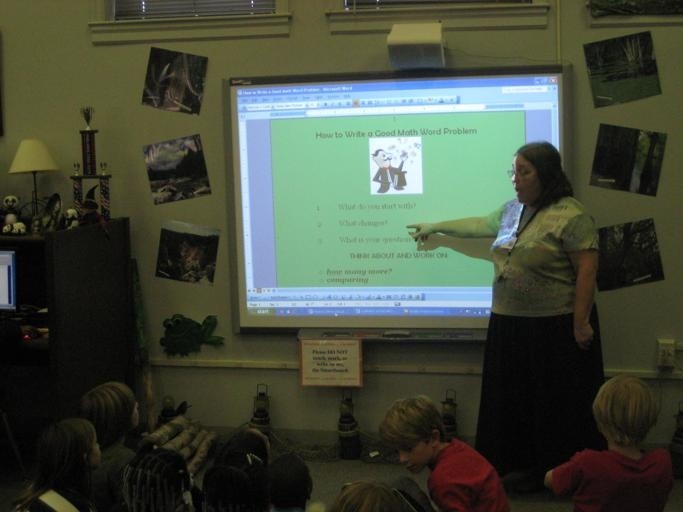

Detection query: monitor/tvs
[{"left": 0, "top": 250, "right": 18, "bottom": 314}]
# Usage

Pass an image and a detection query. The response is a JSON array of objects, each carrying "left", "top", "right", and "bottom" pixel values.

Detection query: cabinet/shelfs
[{"left": 0, "top": 217, "right": 134, "bottom": 466}]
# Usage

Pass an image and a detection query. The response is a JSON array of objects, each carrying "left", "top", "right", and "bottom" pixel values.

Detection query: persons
[
  {"left": 1, "top": 374, "right": 683, "bottom": 512},
  {"left": 406, "top": 141, "right": 608, "bottom": 499}
]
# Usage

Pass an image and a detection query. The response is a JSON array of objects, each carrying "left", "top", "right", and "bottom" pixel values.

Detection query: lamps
[
  {"left": 387, "top": 23, "right": 446, "bottom": 70},
  {"left": 8, "top": 139, "right": 59, "bottom": 215}
]
